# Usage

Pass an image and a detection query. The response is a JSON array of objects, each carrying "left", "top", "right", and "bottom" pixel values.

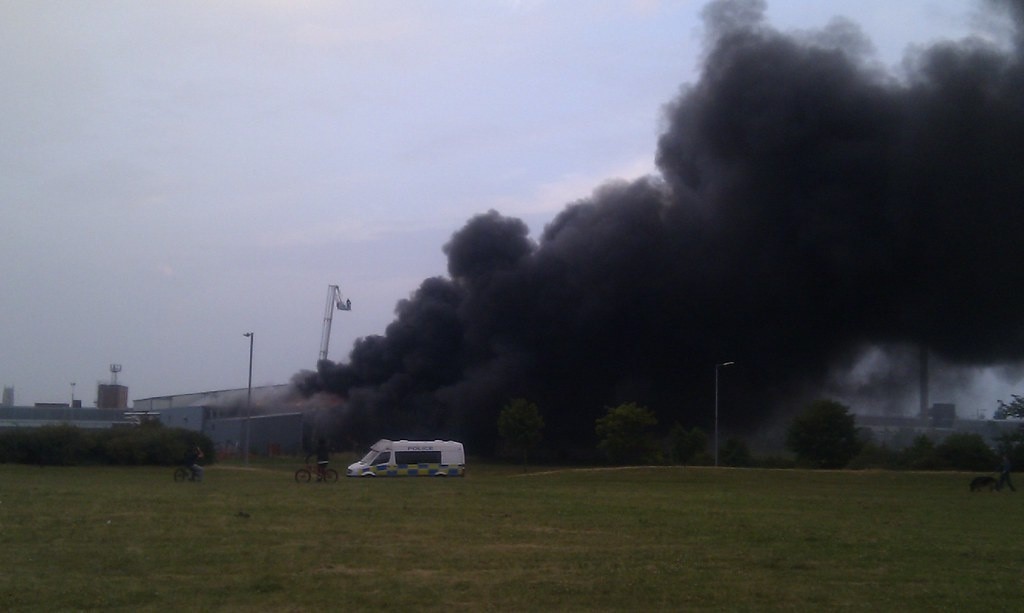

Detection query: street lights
[
  {"left": 715, "top": 360, "right": 736, "bottom": 468},
  {"left": 243, "top": 330, "right": 252, "bottom": 467}
]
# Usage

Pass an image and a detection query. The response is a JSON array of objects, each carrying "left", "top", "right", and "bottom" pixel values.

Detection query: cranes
[{"left": 320, "top": 283, "right": 352, "bottom": 361}]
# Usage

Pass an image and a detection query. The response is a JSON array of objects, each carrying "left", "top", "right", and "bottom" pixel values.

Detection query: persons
[
  {"left": 316, "top": 439, "right": 329, "bottom": 482},
  {"left": 186, "top": 444, "right": 202, "bottom": 481},
  {"left": 994, "top": 456, "right": 1016, "bottom": 492}
]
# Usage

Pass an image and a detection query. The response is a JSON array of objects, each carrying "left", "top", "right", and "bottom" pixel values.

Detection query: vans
[{"left": 345, "top": 438, "right": 467, "bottom": 478}]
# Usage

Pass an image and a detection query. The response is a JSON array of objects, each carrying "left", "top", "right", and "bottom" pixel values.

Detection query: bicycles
[
  {"left": 293, "top": 459, "right": 338, "bottom": 485},
  {"left": 174, "top": 465, "right": 195, "bottom": 483}
]
